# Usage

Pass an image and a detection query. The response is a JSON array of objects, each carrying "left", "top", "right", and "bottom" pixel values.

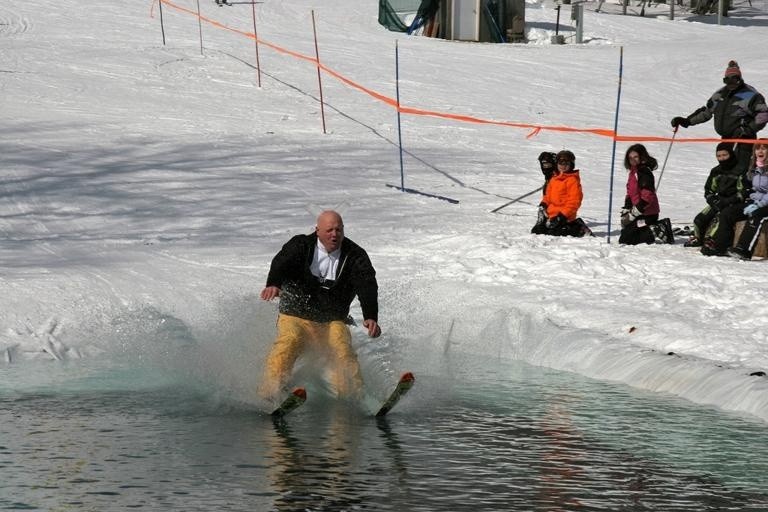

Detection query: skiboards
[
  {"left": 269, "top": 373, "right": 415, "bottom": 419},
  {"left": 672, "top": 226, "right": 695, "bottom": 236}
]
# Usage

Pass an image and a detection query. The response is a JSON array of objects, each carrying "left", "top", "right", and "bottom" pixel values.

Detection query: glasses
[{"left": 723, "top": 77, "right": 741, "bottom": 84}]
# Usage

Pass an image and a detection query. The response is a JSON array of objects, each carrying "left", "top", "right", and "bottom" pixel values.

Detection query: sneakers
[
  {"left": 648, "top": 218, "right": 752, "bottom": 262},
  {"left": 571, "top": 218, "right": 596, "bottom": 237}
]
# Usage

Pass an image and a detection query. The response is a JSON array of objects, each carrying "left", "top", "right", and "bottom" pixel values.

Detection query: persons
[
  {"left": 670, "top": 60, "right": 768, "bottom": 151},
  {"left": 696, "top": 136, "right": 768, "bottom": 261},
  {"left": 537, "top": 151, "right": 560, "bottom": 223},
  {"left": 682, "top": 140, "right": 750, "bottom": 258},
  {"left": 257, "top": 209, "right": 383, "bottom": 414},
  {"left": 538, "top": 150, "right": 584, "bottom": 223},
  {"left": 617, "top": 143, "right": 677, "bottom": 247}
]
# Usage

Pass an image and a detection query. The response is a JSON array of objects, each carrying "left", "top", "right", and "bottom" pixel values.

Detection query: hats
[
  {"left": 538, "top": 150, "right": 575, "bottom": 164},
  {"left": 725, "top": 59, "right": 741, "bottom": 77}
]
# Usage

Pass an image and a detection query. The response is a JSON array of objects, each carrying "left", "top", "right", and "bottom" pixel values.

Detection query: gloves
[
  {"left": 732, "top": 126, "right": 753, "bottom": 139},
  {"left": 703, "top": 193, "right": 729, "bottom": 213},
  {"left": 743, "top": 203, "right": 759, "bottom": 217},
  {"left": 619, "top": 205, "right": 644, "bottom": 228},
  {"left": 671, "top": 117, "right": 689, "bottom": 128},
  {"left": 536, "top": 203, "right": 564, "bottom": 229}
]
